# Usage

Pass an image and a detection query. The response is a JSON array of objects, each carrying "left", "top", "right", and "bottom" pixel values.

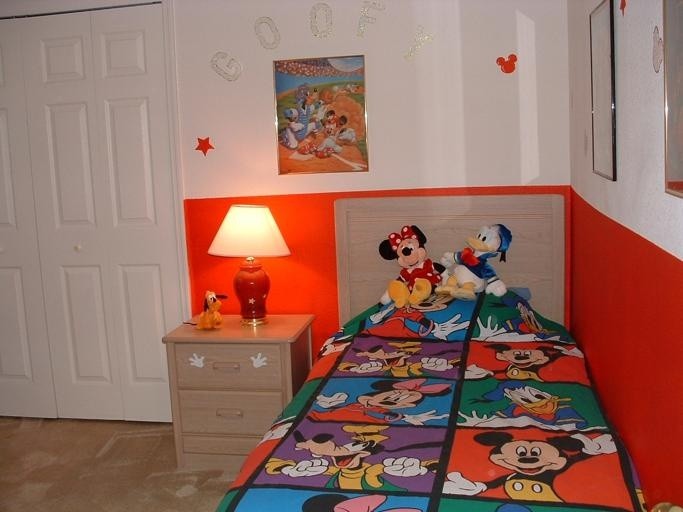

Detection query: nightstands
[{"left": 161, "top": 312, "right": 316, "bottom": 474}]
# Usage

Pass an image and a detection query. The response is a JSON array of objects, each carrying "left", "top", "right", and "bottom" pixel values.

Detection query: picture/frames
[
  {"left": 275, "top": 52, "right": 370, "bottom": 175},
  {"left": 588, "top": 0, "right": 619, "bottom": 182}
]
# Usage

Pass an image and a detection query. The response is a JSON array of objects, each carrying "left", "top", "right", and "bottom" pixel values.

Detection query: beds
[{"left": 217, "top": 193, "right": 648, "bottom": 512}]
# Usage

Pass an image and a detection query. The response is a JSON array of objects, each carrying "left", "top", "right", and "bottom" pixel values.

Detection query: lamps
[{"left": 204, "top": 201, "right": 294, "bottom": 325}]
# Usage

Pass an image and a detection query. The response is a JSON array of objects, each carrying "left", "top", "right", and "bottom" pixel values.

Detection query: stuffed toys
[
  {"left": 195, "top": 290, "right": 228, "bottom": 330},
  {"left": 378, "top": 224, "right": 514, "bottom": 308}
]
